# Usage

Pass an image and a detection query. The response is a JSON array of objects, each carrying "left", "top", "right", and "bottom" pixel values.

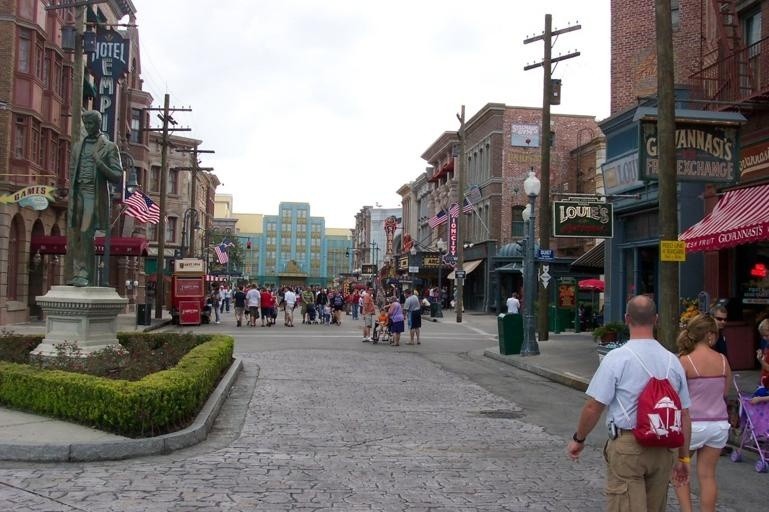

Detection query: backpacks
[{"left": 614, "top": 345, "right": 685, "bottom": 448}]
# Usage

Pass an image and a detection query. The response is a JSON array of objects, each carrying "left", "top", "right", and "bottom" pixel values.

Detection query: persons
[
  {"left": 756, "top": 319, "right": 769, "bottom": 387},
  {"left": 566, "top": 295, "right": 693, "bottom": 512},
  {"left": 505, "top": 291, "right": 521, "bottom": 315},
  {"left": 668, "top": 313, "right": 731, "bottom": 512},
  {"left": 207, "top": 274, "right": 447, "bottom": 349},
  {"left": 750, "top": 396, "right": 769, "bottom": 405},
  {"left": 706, "top": 304, "right": 728, "bottom": 355},
  {"left": 65, "top": 110, "right": 123, "bottom": 286}
]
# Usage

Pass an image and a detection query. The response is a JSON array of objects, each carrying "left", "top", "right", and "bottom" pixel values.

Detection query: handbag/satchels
[{"left": 387, "top": 318, "right": 393, "bottom": 326}]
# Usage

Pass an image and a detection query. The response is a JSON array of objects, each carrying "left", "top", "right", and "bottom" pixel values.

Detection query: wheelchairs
[{"left": 371, "top": 308, "right": 394, "bottom": 344}]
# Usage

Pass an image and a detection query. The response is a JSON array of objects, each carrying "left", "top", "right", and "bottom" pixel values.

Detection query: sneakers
[{"left": 362, "top": 336, "right": 374, "bottom": 342}]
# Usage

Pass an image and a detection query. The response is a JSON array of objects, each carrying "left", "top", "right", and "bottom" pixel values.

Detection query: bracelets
[{"left": 677, "top": 456, "right": 690, "bottom": 463}]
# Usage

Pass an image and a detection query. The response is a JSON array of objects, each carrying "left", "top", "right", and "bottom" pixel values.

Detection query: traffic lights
[
  {"left": 246, "top": 241, "right": 251, "bottom": 249},
  {"left": 345, "top": 252, "right": 349, "bottom": 257}
]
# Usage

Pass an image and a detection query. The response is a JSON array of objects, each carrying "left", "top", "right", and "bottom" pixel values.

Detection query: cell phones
[{"left": 607, "top": 416, "right": 617, "bottom": 440}]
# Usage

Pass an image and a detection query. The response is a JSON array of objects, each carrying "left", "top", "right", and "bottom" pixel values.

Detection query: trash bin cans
[
  {"left": 430, "top": 302, "right": 442, "bottom": 317},
  {"left": 136, "top": 304, "right": 151, "bottom": 324},
  {"left": 498, "top": 313, "right": 524, "bottom": 355},
  {"left": 549, "top": 308, "right": 568, "bottom": 330}
]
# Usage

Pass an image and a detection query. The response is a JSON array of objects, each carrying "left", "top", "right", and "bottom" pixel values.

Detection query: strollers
[
  {"left": 330, "top": 307, "right": 342, "bottom": 326},
  {"left": 304, "top": 303, "right": 319, "bottom": 325},
  {"left": 731, "top": 371, "right": 768, "bottom": 472}
]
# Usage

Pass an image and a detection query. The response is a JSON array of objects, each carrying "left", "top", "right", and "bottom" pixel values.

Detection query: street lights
[
  {"left": 521, "top": 203, "right": 536, "bottom": 352},
  {"left": 521, "top": 167, "right": 542, "bottom": 358},
  {"left": 180, "top": 207, "right": 201, "bottom": 258}
]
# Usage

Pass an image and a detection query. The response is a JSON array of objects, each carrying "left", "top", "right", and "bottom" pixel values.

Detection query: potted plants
[{"left": 592, "top": 321, "right": 630, "bottom": 345}]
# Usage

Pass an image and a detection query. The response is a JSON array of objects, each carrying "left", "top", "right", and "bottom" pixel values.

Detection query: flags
[
  {"left": 121, "top": 188, "right": 169, "bottom": 225},
  {"left": 221, "top": 238, "right": 235, "bottom": 248},
  {"left": 450, "top": 198, "right": 472, "bottom": 217},
  {"left": 427, "top": 210, "right": 448, "bottom": 229},
  {"left": 215, "top": 244, "right": 228, "bottom": 265}
]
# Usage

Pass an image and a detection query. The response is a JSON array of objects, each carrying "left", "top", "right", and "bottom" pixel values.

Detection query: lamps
[{"left": 27, "top": 249, "right": 42, "bottom": 273}]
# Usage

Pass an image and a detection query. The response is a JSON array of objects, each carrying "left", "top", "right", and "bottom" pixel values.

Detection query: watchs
[{"left": 572, "top": 432, "right": 586, "bottom": 443}]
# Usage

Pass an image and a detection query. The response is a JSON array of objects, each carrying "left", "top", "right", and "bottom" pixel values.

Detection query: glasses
[{"left": 714, "top": 316, "right": 728, "bottom": 321}]
